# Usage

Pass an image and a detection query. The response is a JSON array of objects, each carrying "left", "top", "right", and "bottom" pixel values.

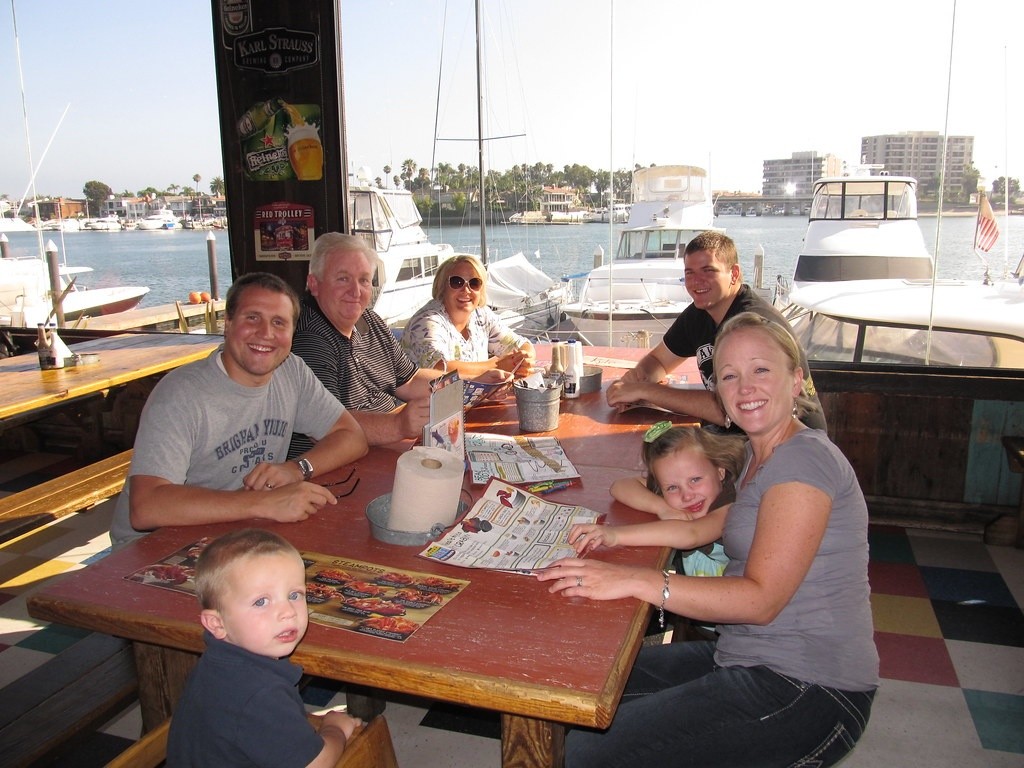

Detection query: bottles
[
  {"left": 38, "top": 323, "right": 51, "bottom": 369},
  {"left": 236, "top": 97, "right": 284, "bottom": 140},
  {"left": 550, "top": 339, "right": 584, "bottom": 398},
  {"left": 49, "top": 324, "right": 64, "bottom": 368}
]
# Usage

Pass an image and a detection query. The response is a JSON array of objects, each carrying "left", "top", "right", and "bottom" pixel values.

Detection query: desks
[
  {"left": 0, "top": 331, "right": 231, "bottom": 429},
  {"left": 32, "top": 342, "right": 725, "bottom": 768}
]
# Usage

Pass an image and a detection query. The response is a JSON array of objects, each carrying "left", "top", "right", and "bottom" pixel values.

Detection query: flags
[{"left": 977, "top": 196, "right": 999, "bottom": 250}]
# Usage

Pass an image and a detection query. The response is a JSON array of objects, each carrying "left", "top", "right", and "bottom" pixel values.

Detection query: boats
[
  {"left": 0, "top": 101, "right": 230, "bottom": 331},
  {"left": 557, "top": 163, "right": 727, "bottom": 350},
  {"left": 771, "top": 153, "right": 1024, "bottom": 369}
]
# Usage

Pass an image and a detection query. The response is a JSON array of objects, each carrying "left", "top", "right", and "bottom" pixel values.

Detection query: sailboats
[{"left": 347, "top": 1, "right": 630, "bottom": 346}]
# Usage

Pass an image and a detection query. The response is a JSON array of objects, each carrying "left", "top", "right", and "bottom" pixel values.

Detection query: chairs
[{"left": 108, "top": 716, "right": 400, "bottom": 768}]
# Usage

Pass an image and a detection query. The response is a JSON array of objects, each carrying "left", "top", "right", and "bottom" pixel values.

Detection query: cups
[{"left": 288, "top": 128, "right": 323, "bottom": 181}]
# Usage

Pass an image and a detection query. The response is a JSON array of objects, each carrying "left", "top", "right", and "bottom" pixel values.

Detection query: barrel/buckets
[
  {"left": 514, "top": 378, "right": 564, "bottom": 432},
  {"left": 366, "top": 488, "right": 474, "bottom": 548}
]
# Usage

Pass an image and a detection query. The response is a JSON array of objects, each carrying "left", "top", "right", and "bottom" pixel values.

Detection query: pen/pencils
[
  {"left": 511, "top": 378, "right": 551, "bottom": 390},
  {"left": 524, "top": 480, "right": 577, "bottom": 495}
]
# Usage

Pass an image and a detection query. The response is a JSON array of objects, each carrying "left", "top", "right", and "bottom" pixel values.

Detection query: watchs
[{"left": 293, "top": 457, "right": 313, "bottom": 480}]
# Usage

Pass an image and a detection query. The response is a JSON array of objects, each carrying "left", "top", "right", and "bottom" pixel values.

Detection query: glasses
[{"left": 446, "top": 275, "right": 484, "bottom": 292}]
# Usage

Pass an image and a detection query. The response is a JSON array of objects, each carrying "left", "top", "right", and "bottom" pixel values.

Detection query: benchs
[
  {"left": 0, "top": 623, "right": 138, "bottom": 768},
  {"left": 0, "top": 444, "right": 133, "bottom": 545}
]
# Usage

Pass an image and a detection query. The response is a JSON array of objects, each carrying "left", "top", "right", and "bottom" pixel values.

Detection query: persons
[
  {"left": 607, "top": 232, "right": 827, "bottom": 434},
  {"left": 399, "top": 254, "right": 535, "bottom": 376},
  {"left": 539, "top": 311, "right": 879, "bottom": 768},
  {"left": 610, "top": 421, "right": 748, "bottom": 641},
  {"left": 289, "top": 231, "right": 515, "bottom": 464},
  {"left": 111, "top": 271, "right": 369, "bottom": 549},
  {"left": 165, "top": 527, "right": 362, "bottom": 768}
]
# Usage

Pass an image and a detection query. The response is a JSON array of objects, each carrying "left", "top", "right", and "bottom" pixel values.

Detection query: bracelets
[{"left": 658, "top": 569, "right": 670, "bottom": 627}]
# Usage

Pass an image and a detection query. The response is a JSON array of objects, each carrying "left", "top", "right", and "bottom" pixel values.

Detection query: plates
[{"left": 64, "top": 353, "right": 99, "bottom": 367}]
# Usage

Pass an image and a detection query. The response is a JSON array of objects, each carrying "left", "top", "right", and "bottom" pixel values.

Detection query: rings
[
  {"left": 577, "top": 577, "right": 583, "bottom": 586},
  {"left": 266, "top": 484, "right": 272, "bottom": 488}
]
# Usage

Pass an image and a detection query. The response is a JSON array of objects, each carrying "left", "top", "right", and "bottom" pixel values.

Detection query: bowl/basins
[{"left": 580, "top": 364, "right": 603, "bottom": 394}]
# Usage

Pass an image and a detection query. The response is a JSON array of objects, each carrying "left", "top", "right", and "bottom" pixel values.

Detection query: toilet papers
[{"left": 386, "top": 445, "right": 465, "bottom": 531}]
